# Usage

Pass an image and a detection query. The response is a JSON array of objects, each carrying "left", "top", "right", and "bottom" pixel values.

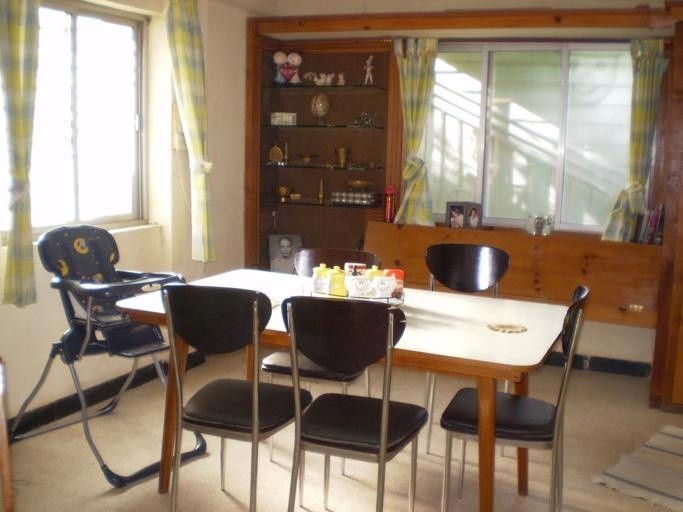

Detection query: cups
[{"left": 334, "top": 147, "right": 350, "bottom": 167}]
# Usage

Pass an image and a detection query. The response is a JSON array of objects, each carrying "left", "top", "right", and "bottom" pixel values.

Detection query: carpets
[{"left": 592, "top": 422, "right": 681, "bottom": 509}]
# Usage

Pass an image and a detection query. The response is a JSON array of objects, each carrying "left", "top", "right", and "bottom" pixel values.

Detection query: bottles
[
  {"left": 318, "top": 180, "right": 324, "bottom": 199},
  {"left": 268, "top": 141, "right": 282, "bottom": 163},
  {"left": 311, "top": 262, "right": 404, "bottom": 301}
]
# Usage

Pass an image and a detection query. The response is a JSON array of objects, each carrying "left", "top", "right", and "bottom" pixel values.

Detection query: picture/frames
[{"left": 445, "top": 201, "right": 483, "bottom": 229}]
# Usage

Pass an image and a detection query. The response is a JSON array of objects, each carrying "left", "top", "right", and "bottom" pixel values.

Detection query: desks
[{"left": 109, "top": 268, "right": 571, "bottom": 512}]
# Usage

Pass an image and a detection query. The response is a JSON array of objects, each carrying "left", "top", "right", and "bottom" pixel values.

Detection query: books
[{"left": 632, "top": 203, "right": 664, "bottom": 245}]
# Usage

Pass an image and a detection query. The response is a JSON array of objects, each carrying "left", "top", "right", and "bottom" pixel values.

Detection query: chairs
[
  {"left": 423, "top": 239, "right": 512, "bottom": 457},
  {"left": 160, "top": 279, "right": 307, "bottom": 511},
  {"left": 262, "top": 246, "right": 374, "bottom": 480},
  {"left": 437, "top": 282, "right": 598, "bottom": 512},
  {"left": 278, "top": 291, "right": 430, "bottom": 511},
  {"left": 10, "top": 221, "right": 206, "bottom": 488}
]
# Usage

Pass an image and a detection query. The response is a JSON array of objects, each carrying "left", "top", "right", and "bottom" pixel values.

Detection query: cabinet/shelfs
[
  {"left": 363, "top": 221, "right": 432, "bottom": 289},
  {"left": 259, "top": 82, "right": 392, "bottom": 210},
  {"left": 433, "top": 222, "right": 497, "bottom": 299},
  {"left": 497, "top": 228, "right": 661, "bottom": 328}
]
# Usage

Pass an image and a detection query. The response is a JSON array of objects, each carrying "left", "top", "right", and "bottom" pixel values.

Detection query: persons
[
  {"left": 533, "top": 217, "right": 545, "bottom": 236},
  {"left": 450, "top": 206, "right": 465, "bottom": 229},
  {"left": 468, "top": 207, "right": 481, "bottom": 229},
  {"left": 270, "top": 236, "right": 296, "bottom": 275}
]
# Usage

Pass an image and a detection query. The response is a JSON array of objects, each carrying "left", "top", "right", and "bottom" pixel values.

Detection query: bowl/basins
[
  {"left": 345, "top": 180, "right": 369, "bottom": 190},
  {"left": 272, "top": 185, "right": 292, "bottom": 197},
  {"left": 289, "top": 194, "right": 301, "bottom": 201}
]
